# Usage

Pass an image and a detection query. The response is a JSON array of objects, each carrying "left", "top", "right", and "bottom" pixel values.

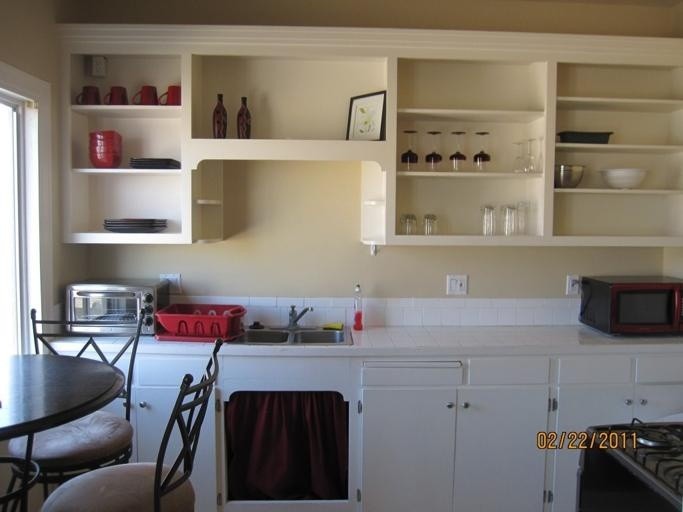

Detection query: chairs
[{"left": 2, "top": 306, "right": 146, "bottom": 510}]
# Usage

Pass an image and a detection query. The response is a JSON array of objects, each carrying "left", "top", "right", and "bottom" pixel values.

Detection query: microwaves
[{"left": 577, "top": 275, "right": 683, "bottom": 335}]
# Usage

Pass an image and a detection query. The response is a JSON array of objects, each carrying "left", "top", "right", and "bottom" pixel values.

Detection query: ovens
[{"left": 64, "top": 277, "right": 169, "bottom": 337}]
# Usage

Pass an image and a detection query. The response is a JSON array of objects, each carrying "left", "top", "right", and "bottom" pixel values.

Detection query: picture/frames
[{"left": 345, "top": 89, "right": 386, "bottom": 142}]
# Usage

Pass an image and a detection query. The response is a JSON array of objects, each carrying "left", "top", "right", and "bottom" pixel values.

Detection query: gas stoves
[{"left": 573, "top": 415, "right": 682, "bottom": 511}]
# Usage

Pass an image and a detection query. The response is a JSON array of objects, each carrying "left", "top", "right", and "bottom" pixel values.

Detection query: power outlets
[{"left": 565, "top": 271, "right": 578, "bottom": 295}]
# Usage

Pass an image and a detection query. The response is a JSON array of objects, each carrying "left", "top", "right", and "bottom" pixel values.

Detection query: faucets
[{"left": 289, "top": 306, "right": 314, "bottom": 329}]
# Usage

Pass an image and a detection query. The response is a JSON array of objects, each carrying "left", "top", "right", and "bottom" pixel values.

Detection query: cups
[
  {"left": 516, "top": 201, "right": 531, "bottom": 235},
  {"left": 157, "top": 84, "right": 181, "bottom": 106},
  {"left": 480, "top": 206, "right": 497, "bottom": 236},
  {"left": 500, "top": 206, "right": 516, "bottom": 236},
  {"left": 399, "top": 213, "right": 417, "bottom": 235},
  {"left": 130, "top": 83, "right": 157, "bottom": 106},
  {"left": 75, "top": 84, "right": 99, "bottom": 105},
  {"left": 471, "top": 132, "right": 493, "bottom": 172},
  {"left": 423, "top": 214, "right": 438, "bottom": 235},
  {"left": 102, "top": 86, "right": 129, "bottom": 104},
  {"left": 400, "top": 130, "right": 418, "bottom": 172},
  {"left": 425, "top": 130, "right": 442, "bottom": 171},
  {"left": 450, "top": 130, "right": 468, "bottom": 173}
]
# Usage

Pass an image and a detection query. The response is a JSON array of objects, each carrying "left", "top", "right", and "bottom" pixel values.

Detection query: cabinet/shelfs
[
  {"left": 359, "top": 358, "right": 551, "bottom": 511},
  {"left": 52, "top": 23, "right": 682, "bottom": 252},
  {"left": 549, "top": 347, "right": 683, "bottom": 511}
]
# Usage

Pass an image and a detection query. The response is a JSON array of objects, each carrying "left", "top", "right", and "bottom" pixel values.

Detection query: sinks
[
  {"left": 292, "top": 331, "right": 347, "bottom": 352},
  {"left": 227, "top": 330, "right": 291, "bottom": 354}
]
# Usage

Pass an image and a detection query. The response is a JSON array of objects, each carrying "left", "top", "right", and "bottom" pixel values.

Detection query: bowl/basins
[
  {"left": 556, "top": 164, "right": 585, "bottom": 189},
  {"left": 600, "top": 168, "right": 647, "bottom": 189},
  {"left": 87, "top": 130, "right": 122, "bottom": 168}
]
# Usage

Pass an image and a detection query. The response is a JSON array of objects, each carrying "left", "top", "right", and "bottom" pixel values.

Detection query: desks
[{"left": 1, "top": 351, "right": 128, "bottom": 511}]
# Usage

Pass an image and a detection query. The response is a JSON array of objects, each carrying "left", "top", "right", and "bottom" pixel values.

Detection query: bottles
[
  {"left": 236, "top": 96, "right": 250, "bottom": 139},
  {"left": 211, "top": 93, "right": 226, "bottom": 139}
]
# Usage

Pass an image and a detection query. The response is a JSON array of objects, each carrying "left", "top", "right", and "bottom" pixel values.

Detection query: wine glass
[{"left": 511, "top": 139, "right": 537, "bottom": 173}]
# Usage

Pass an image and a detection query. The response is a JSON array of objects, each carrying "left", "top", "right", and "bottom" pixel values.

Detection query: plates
[
  {"left": 128, "top": 158, "right": 181, "bottom": 169},
  {"left": 103, "top": 219, "right": 167, "bottom": 234},
  {"left": 557, "top": 130, "right": 613, "bottom": 145}
]
